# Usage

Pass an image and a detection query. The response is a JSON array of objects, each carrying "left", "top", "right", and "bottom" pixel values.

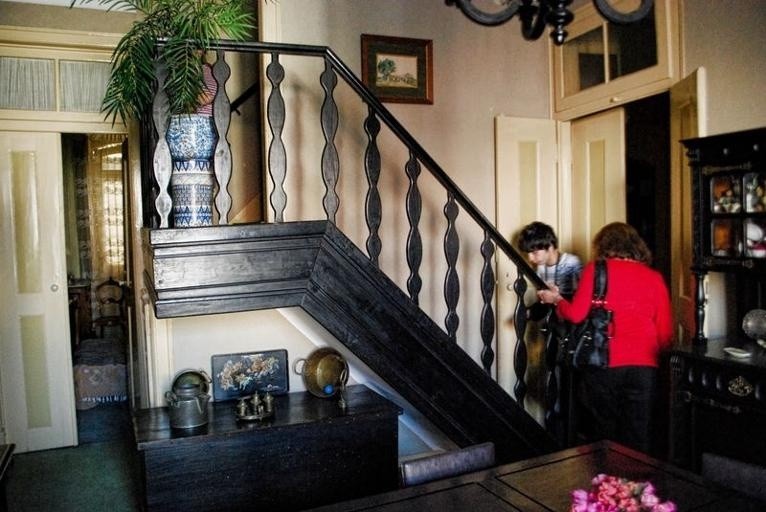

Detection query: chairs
[
  {"left": 90, "top": 277, "right": 125, "bottom": 338},
  {"left": 400, "top": 443, "right": 496, "bottom": 487}
]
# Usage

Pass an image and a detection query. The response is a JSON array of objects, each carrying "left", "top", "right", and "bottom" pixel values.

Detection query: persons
[
  {"left": 517, "top": 221, "right": 587, "bottom": 425},
  {"left": 539, "top": 223, "right": 674, "bottom": 455}
]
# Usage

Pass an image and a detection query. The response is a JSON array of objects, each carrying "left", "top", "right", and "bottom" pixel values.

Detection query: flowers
[{"left": 566, "top": 471, "right": 677, "bottom": 512}]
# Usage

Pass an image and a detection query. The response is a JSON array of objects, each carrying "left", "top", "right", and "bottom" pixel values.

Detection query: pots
[
  {"left": 294, "top": 347, "right": 349, "bottom": 398},
  {"left": 164, "top": 370, "right": 213, "bottom": 402}
]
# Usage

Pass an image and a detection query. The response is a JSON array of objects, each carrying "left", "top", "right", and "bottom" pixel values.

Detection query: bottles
[{"left": 165, "top": 384, "right": 209, "bottom": 429}]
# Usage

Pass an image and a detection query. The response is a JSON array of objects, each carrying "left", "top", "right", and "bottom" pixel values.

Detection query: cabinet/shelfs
[{"left": 662, "top": 126, "right": 766, "bottom": 471}]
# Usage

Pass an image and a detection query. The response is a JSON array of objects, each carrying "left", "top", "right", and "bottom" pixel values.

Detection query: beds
[{"left": 73, "top": 339, "right": 128, "bottom": 410}]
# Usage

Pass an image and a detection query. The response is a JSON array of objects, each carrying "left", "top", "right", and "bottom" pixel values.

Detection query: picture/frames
[
  {"left": 356, "top": 32, "right": 434, "bottom": 105},
  {"left": 212, "top": 350, "right": 291, "bottom": 403}
]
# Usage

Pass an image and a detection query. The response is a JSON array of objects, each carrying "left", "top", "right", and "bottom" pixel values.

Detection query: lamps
[{"left": 444, "top": 0, "right": 658, "bottom": 45}]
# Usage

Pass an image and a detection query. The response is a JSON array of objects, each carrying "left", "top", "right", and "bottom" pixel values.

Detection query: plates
[{"left": 724, "top": 347, "right": 752, "bottom": 359}]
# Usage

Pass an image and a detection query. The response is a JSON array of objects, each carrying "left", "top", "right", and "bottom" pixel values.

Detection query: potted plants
[{"left": 65, "top": 1, "right": 268, "bottom": 230}]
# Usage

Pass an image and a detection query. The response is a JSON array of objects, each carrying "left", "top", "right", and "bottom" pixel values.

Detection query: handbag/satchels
[{"left": 561, "top": 259, "right": 610, "bottom": 369}]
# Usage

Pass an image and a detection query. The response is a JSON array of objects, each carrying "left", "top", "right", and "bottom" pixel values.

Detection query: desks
[
  {"left": 130, "top": 383, "right": 404, "bottom": 511},
  {"left": 303, "top": 438, "right": 765, "bottom": 512}
]
